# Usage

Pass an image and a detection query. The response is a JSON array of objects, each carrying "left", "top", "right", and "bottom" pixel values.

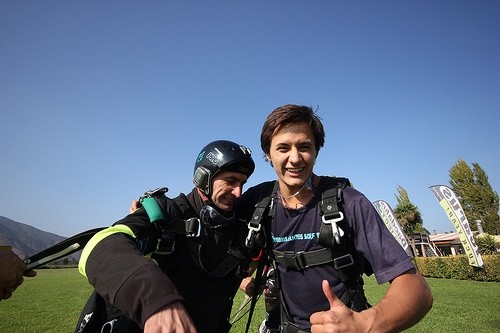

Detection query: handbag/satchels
[{"left": 74, "top": 287, "right": 142, "bottom": 333}]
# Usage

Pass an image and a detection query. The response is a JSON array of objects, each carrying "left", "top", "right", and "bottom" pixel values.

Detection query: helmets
[{"left": 192, "top": 139, "right": 255, "bottom": 197}]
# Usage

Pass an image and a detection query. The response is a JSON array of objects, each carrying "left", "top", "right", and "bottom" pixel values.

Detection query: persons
[
  {"left": 129, "top": 105, "right": 433, "bottom": 333},
  {"left": 73, "top": 140, "right": 257, "bottom": 333}
]
